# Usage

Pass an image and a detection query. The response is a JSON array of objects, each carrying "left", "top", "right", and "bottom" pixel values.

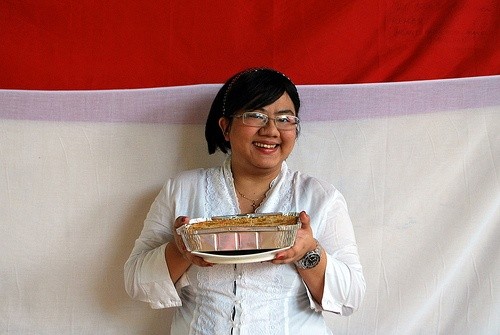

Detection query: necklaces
[{"left": 235, "top": 181, "right": 266, "bottom": 211}]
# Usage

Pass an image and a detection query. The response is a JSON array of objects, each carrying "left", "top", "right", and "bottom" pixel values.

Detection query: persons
[{"left": 124, "top": 67, "right": 366, "bottom": 335}]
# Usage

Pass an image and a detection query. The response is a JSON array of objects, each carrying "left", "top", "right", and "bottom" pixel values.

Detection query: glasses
[{"left": 234, "top": 112, "right": 300, "bottom": 129}]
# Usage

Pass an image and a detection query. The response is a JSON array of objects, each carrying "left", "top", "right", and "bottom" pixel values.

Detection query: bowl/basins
[{"left": 176, "top": 212, "right": 303, "bottom": 252}]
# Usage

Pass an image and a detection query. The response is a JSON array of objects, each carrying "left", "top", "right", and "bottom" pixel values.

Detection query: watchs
[{"left": 293, "top": 238, "right": 323, "bottom": 270}]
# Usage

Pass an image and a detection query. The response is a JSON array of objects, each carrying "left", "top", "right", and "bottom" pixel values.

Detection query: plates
[{"left": 190, "top": 247, "right": 292, "bottom": 264}]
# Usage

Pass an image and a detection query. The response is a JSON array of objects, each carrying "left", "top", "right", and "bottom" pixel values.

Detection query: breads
[{"left": 186, "top": 215, "right": 297, "bottom": 247}]
